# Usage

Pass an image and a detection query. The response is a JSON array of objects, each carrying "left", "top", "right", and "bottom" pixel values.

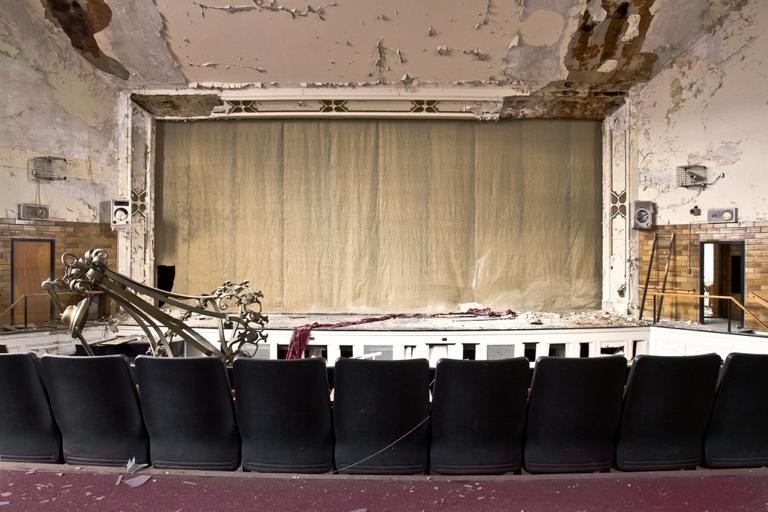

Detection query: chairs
[
  {"left": 524, "top": 356, "right": 630, "bottom": 476},
  {"left": 1, "top": 352, "right": 60, "bottom": 466},
  {"left": 431, "top": 357, "right": 531, "bottom": 475},
  {"left": 333, "top": 357, "right": 432, "bottom": 475},
  {"left": 133, "top": 356, "right": 241, "bottom": 473},
  {"left": 234, "top": 356, "right": 336, "bottom": 476},
  {"left": 704, "top": 354, "right": 768, "bottom": 468},
  {"left": 38, "top": 356, "right": 150, "bottom": 467},
  {"left": 617, "top": 355, "right": 724, "bottom": 473}
]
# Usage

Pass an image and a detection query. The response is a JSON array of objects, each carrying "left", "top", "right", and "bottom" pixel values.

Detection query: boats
[
  {"left": 99, "top": 199, "right": 132, "bottom": 233},
  {"left": 633, "top": 200, "right": 653, "bottom": 230},
  {"left": 18, "top": 204, "right": 50, "bottom": 220},
  {"left": 707, "top": 207, "right": 737, "bottom": 223}
]
